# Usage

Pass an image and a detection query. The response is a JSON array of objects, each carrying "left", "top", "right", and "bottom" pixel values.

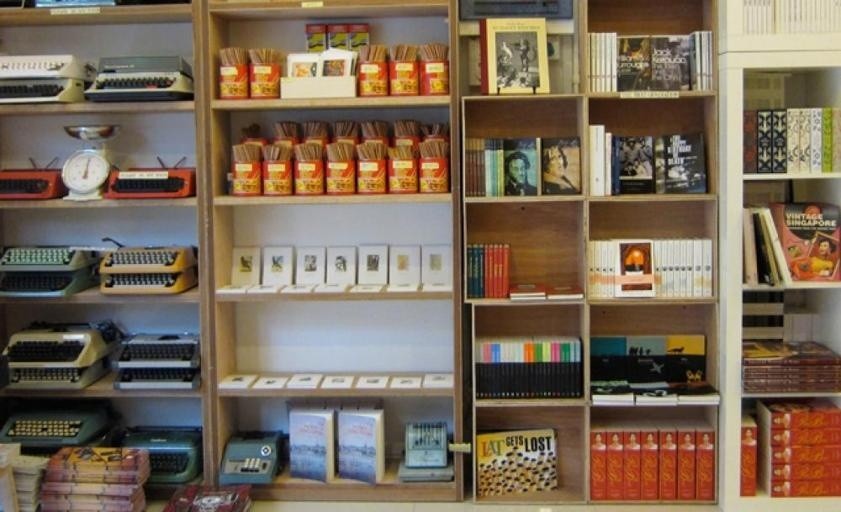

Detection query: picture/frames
[{"left": 485, "top": 18, "right": 550, "bottom": 94}]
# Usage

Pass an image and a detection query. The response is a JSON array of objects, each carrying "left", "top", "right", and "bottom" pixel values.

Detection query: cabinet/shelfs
[
  {"left": 205, "top": 0, "right": 465, "bottom": 502},
  {"left": 0, "top": 2, "right": 214, "bottom": 493},
  {"left": 717, "top": 5, "right": 841, "bottom": 511}
]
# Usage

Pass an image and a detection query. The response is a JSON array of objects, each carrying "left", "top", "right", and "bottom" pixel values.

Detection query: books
[
  {"left": 0, "top": 441, "right": 151, "bottom": 511},
  {"left": 161, "top": 482, "right": 253, "bottom": 511},
  {"left": 740, "top": 397, "right": 841, "bottom": 498},
  {"left": 467, "top": 1, "right": 714, "bottom": 93},
  {"left": 590, "top": 417, "right": 715, "bottom": 506},
  {"left": 587, "top": 240, "right": 722, "bottom": 405},
  {"left": 743, "top": 200, "right": 841, "bottom": 288},
  {"left": 744, "top": 107, "right": 834, "bottom": 175},
  {"left": 475, "top": 429, "right": 557, "bottom": 498},
  {"left": 208, "top": 240, "right": 454, "bottom": 391},
  {"left": 740, "top": 299, "right": 841, "bottom": 394},
  {"left": 282, "top": 397, "right": 388, "bottom": 486},
  {"left": 464, "top": 134, "right": 709, "bottom": 201},
  {"left": 467, "top": 245, "right": 585, "bottom": 400}
]
